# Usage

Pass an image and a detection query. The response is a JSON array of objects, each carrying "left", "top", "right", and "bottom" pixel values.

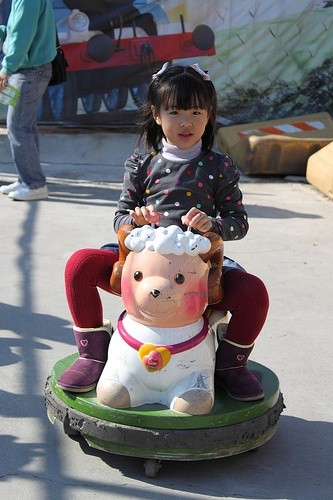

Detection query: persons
[
  {"left": 57, "top": 62, "right": 269, "bottom": 402},
  {"left": 0, "top": 0, "right": 57, "bottom": 200}
]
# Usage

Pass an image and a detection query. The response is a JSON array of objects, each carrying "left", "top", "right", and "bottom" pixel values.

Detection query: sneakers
[{"left": 0, "top": 182, "right": 50, "bottom": 200}]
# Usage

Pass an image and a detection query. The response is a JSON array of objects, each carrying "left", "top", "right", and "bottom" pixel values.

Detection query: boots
[
  {"left": 214, "top": 323, "right": 265, "bottom": 402},
  {"left": 56, "top": 319, "right": 114, "bottom": 393}
]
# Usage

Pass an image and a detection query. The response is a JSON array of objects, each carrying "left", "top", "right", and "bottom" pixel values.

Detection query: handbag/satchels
[{"left": 49, "top": 24, "right": 69, "bottom": 87}]
